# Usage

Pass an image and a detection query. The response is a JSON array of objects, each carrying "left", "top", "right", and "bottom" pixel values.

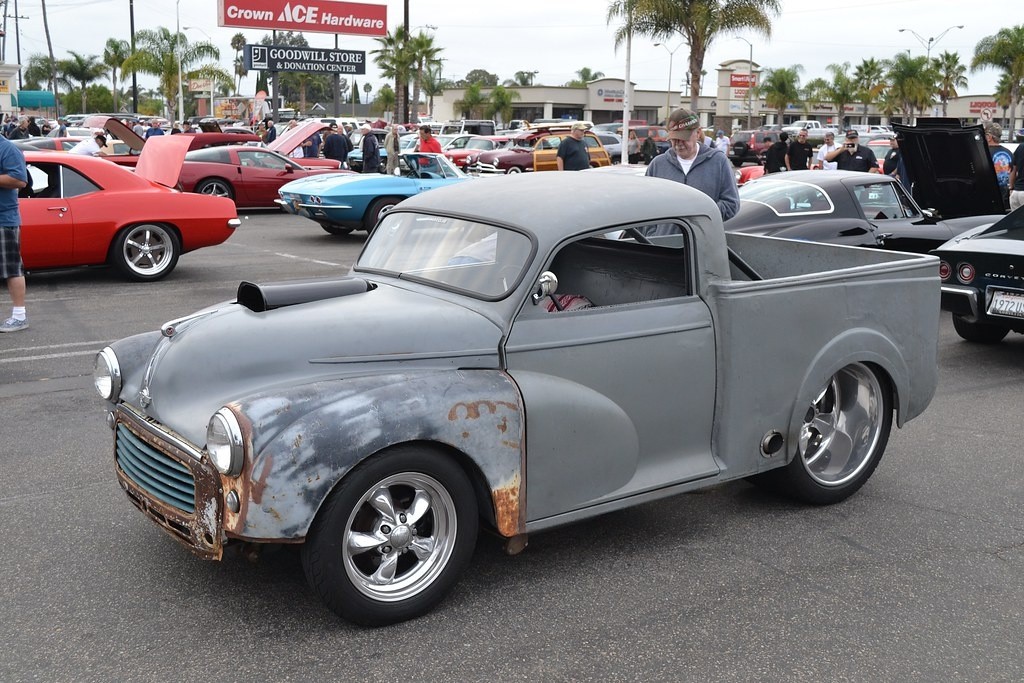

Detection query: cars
[
  {"left": 273, "top": 147, "right": 480, "bottom": 234},
  {"left": 718, "top": 114, "right": 1013, "bottom": 254},
  {"left": 768, "top": 119, "right": 1024, "bottom": 174},
  {"left": 22, "top": 152, "right": 240, "bottom": 280},
  {"left": 930, "top": 203, "right": 1024, "bottom": 354},
  {"left": 178, "top": 146, "right": 361, "bottom": 213},
  {"left": 10, "top": 109, "right": 715, "bottom": 190}
]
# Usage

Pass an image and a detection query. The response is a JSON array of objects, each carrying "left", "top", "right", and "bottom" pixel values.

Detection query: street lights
[
  {"left": 409, "top": 24, "right": 438, "bottom": 36},
  {"left": 899, "top": 25, "right": 965, "bottom": 70},
  {"left": 654, "top": 41, "right": 691, "bottom": 127},
  {"left": 531, "top": 70, "right": 539, "bottom": 87},
  {"left": 183, "top": 25, "right": 217, "bottom": 116},
  {"left": 735, "top": 35, "right": 753, "bottom": 129}
]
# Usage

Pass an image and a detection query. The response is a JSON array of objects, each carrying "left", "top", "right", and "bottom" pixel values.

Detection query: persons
[
  {"left": 418, "top": 125, "right": 442, "bottom": 179},
  {"left": 359, "top": 124, "right": 381, "bottom": 173},
  {"left": 249, "top": 113, "right": 353, "bottom": 170},
  {"left": 69, "top": 133, "right": 107, "bottom": 156},
  {"left": 4, "top": 113, "right": 70, "bottom": 139},
  {"left": 0, "top": 132, "right": 29, "bottom": 332},
  {"left": 384, "top": 125, "right": 400, "bottom": 175},
  {"left": 557, "top": 122, "right": 592, "bottom": 171},
  {"left": 123, "top": 117, "right": 196, "bottom": 140},
  {"left": 637, "top": 108, "right": 740, "bottom": 235},
  {"left": 627, "top": 122, "right": 1024, "bottom": 213}
]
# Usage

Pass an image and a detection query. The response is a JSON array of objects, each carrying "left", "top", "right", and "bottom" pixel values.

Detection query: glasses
[
  {"left": 824, "top": 138, "right": 831, "bottom": 141},
  {"left": 799, "top": 134, "right": 806, "bottom": 137}
]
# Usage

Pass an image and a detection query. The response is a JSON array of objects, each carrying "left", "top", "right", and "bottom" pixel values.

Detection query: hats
[
  {"left": 360, "top": 124, "right": 371, "bottom": 132},
  {"left": 716, "top": 130, "right": 724, "bottom": 136},
  {"left": 329, "top": 122, "right": 338, "bottom": 128},
  {"left": 666, "top": 107, "right": 700, "bottom": 141},
  {"left": 985, "top": 123, "right": 1002, "bottom": 137},
  {"left": 888, "top": 134, "right": 897, "bottom": 140},
  {"left": 183, "top": 121, "right": 189, "bottom": 124},
  {"left": 571, "top": 122, "right": 588, "bottom": 130},
  {"left": 845, "top": 130, "right": 858, "bottom": 139},
  {"left": 97, "top": 135, "right": 108, "bottom": 147}
]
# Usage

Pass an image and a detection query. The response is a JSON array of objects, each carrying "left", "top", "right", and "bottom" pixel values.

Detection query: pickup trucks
[{"left": 97, "top": 166, "right": 941, "bottom": 626}]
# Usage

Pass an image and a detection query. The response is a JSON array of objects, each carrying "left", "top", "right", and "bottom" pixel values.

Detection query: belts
[{"left": 421, "top": 165, "right": 434, "bottom": 167}]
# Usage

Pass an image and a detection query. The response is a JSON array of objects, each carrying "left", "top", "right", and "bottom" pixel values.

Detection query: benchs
[{"left": 511, "top": 235, "right": 685, "bottom": 308}]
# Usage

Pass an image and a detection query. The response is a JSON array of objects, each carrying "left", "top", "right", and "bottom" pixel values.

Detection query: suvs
[
  {"left": 728, "top": 129, "right": 795, "bottom": 167},
  {"left": 468, "top": 122, "right": 612, "bottom": 174}
]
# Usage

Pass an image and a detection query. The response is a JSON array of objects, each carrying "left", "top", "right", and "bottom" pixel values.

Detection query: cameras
[{"left": 846, "top": 143, "right": 855, "bottom": 148}]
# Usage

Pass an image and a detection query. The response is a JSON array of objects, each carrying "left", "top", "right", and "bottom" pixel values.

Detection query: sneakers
[{"left": 0, "top": 318, "right": 28, "bottom": 332}]
